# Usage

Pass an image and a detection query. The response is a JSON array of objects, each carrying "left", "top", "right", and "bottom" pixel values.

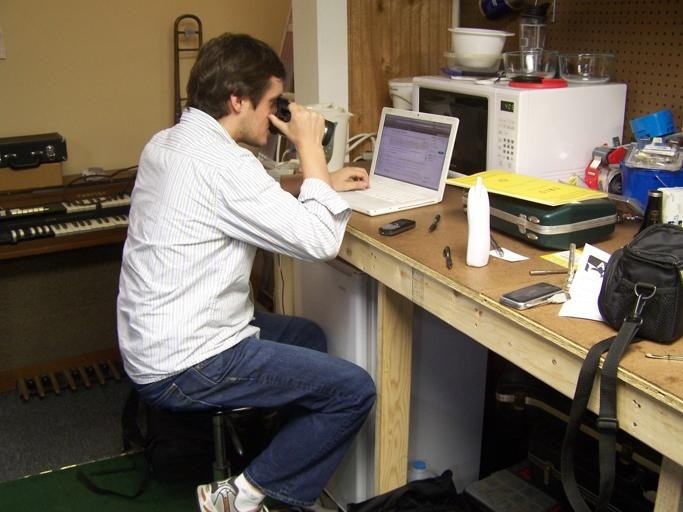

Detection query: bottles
[
  {"left": 406, "top": 460, "right": 438, "bottom": 482},
  {"left": 465, "top": 175, "right": 493, "bottom": 269},
  {"left": 640, "top": 190, "right": 663, "bottom": 232}
]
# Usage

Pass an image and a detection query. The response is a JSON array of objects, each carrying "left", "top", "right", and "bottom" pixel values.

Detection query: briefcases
[{"left": 461, "top": 189, "right": 618, "bottom": 250}]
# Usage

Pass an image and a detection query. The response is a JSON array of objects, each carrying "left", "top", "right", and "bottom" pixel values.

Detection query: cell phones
[
  {"left": 378, "top": 217, "right": 417, "bottom": 235},
  {"left": 500, "top": 283, "right": 564, "bottom": 311}
]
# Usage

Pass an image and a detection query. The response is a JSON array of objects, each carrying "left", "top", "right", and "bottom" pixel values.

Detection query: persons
[{"left": 115, "top": 29, "right": 370, "bottom": 512}]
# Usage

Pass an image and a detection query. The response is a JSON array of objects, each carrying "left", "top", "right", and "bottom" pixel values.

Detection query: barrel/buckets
[{"left": 386, "top": 77, "right": 413, "bottom": 111}]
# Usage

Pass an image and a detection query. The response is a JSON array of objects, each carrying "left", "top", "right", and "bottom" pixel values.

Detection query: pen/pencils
[
  {"left": 491, "top": 236, "right": 504, "bottom": 257},
  {"left": 529, "top": 243, "right": 576, "bottom": 284},
  {"left": 428, "top": 215, "right": 452, "bottom": 270}
]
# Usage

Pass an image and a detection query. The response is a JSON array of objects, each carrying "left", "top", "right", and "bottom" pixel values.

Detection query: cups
[
  {"left": 517, "top": 22, "right": 549, "bottom": 71},
  {"left": 268, "top": 96, "right": 336, "bottom": 148}
]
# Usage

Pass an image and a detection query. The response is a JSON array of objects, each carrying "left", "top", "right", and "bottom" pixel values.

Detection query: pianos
[{"left": 2, "top": 176, "right": 135, "bottom": 261}]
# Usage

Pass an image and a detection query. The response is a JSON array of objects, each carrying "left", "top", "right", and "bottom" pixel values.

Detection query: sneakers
[{"left": 196, "top": 476, "right": 268, "bottom": 512}]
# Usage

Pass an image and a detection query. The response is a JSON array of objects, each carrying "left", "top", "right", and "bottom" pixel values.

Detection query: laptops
[{"left": 332, "top": 107, "right": 460, "bottom": 218}]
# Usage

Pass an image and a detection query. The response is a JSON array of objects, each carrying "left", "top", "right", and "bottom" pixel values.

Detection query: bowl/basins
[
  {"left": 444, "top": 25, "right": 514, "bottom": 66},
  {"left": 502, "top": 49, "right": 559, "bottom": 81},
  {"left": 558, "top": 52, "right": 617, "bottom": 83},
  {"left": 441, "top": 49, "right": 502, "bottom": 75}
]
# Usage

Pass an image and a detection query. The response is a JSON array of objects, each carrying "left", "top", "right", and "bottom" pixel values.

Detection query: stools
[{"left": 182, "top": 405, "right": 257, "bottom": 485}]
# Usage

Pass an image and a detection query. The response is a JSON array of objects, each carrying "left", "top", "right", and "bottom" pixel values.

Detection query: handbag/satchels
[
  {"left": 0, "top": 132, "right": 67, "bottom": 170},
  {"left": 122, "top": 388, "right": 215, "bottom": 483},
  {"left": 597, "top": 221, "right": 683, "bottom": 345}
]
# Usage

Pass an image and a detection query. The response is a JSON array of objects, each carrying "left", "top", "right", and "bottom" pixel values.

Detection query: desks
[{"left": 276, "top": 176, "right": 683, "bottom": 512}]
